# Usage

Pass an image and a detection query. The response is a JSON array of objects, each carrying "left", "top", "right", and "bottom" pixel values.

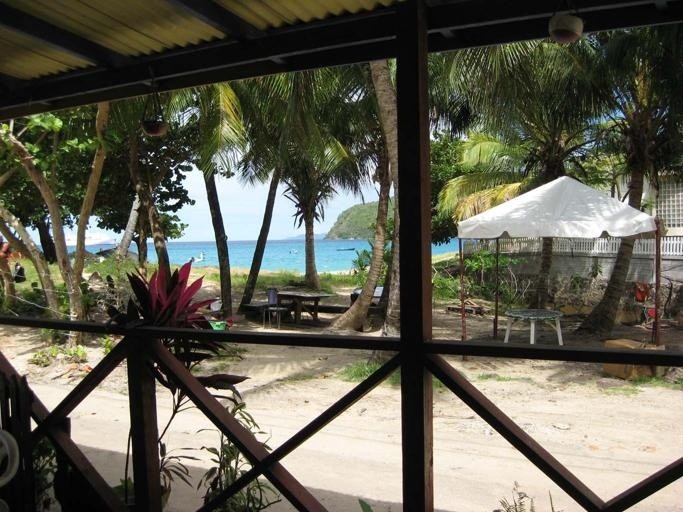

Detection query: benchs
[{"left": 242, "top": 302, "right": 378, "bottom": 332}]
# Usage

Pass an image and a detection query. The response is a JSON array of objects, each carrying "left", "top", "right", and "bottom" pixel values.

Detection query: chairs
[{"left": 262, "top": 288, "right": 289, "bottom": 329}]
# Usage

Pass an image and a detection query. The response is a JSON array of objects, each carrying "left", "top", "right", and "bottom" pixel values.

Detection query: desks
[
  {"left": 276, "top": 293, "right": 330, "bottom": 324},
  {"left": 503, "top": 309, "right": 563, "bottom": 345}
]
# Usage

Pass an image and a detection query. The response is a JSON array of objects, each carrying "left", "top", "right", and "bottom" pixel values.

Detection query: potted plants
[{"left": 107, "top": 258, "right": 250, "bottom": 512}]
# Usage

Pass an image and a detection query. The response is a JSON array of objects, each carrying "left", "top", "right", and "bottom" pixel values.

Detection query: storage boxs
[{"left": 604, "top": 339, "right": 666, "bottom": 380}]
